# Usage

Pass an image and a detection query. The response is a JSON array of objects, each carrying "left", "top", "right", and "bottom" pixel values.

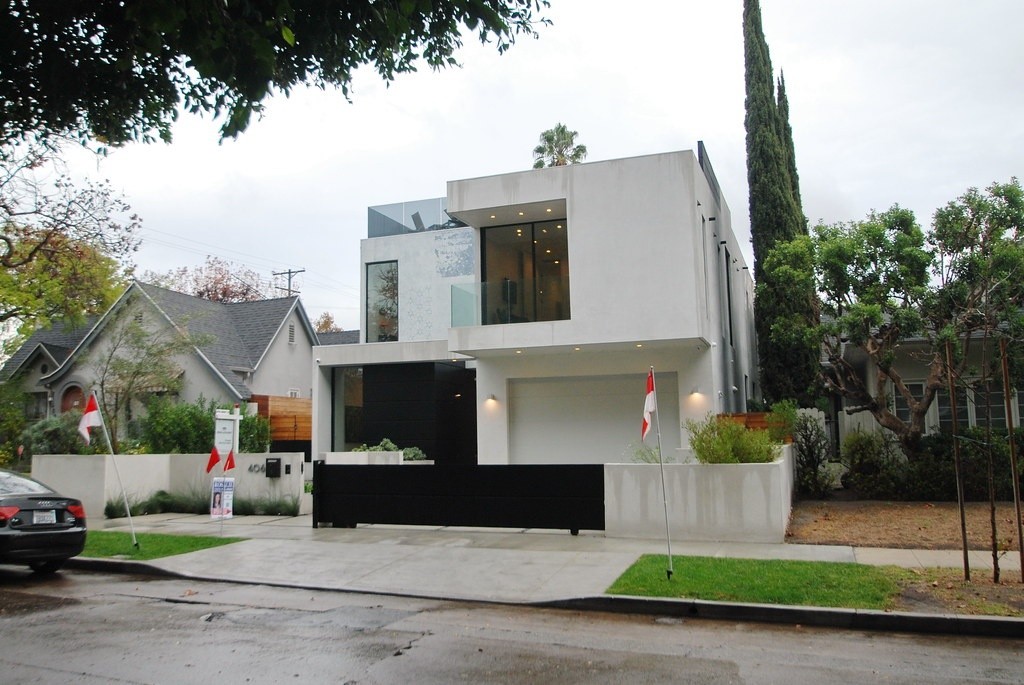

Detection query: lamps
[
  {"left": 709, "top": 217, "right": 715, "bottom": 221},
  {"left": 743, "top": 266, "right": 749, "bottom": 269},
  {"left": 722, "top": 240, "right": 726, "bottom": 244},
  {"left": 487, "top": 394, "right": 494, "bottom": 400},
  {"left": 692, "top": 386, "right": 698, "bottom": 394}
]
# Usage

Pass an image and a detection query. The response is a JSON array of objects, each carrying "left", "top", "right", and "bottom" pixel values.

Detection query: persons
[{"left": 213, "top": 492, "right": 221, "bottom": 515}]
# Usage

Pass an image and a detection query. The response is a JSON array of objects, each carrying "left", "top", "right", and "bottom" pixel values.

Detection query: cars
[{"left": 0, "top": 468, "right": 88, "bottom": 575}]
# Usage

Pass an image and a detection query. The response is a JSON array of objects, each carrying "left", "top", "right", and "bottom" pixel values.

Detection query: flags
[
  {"left": 224, "top": 450, "right": 235, "bottom": 470},
  {"left": 641, "top": 368, "right": 657, "bottom": 441},
  {"left": 206, "top": 446, "right": 221, "bottom": 474},
  {"left": 77, "top": 395, "right": 102, "bottom": 446}
]
